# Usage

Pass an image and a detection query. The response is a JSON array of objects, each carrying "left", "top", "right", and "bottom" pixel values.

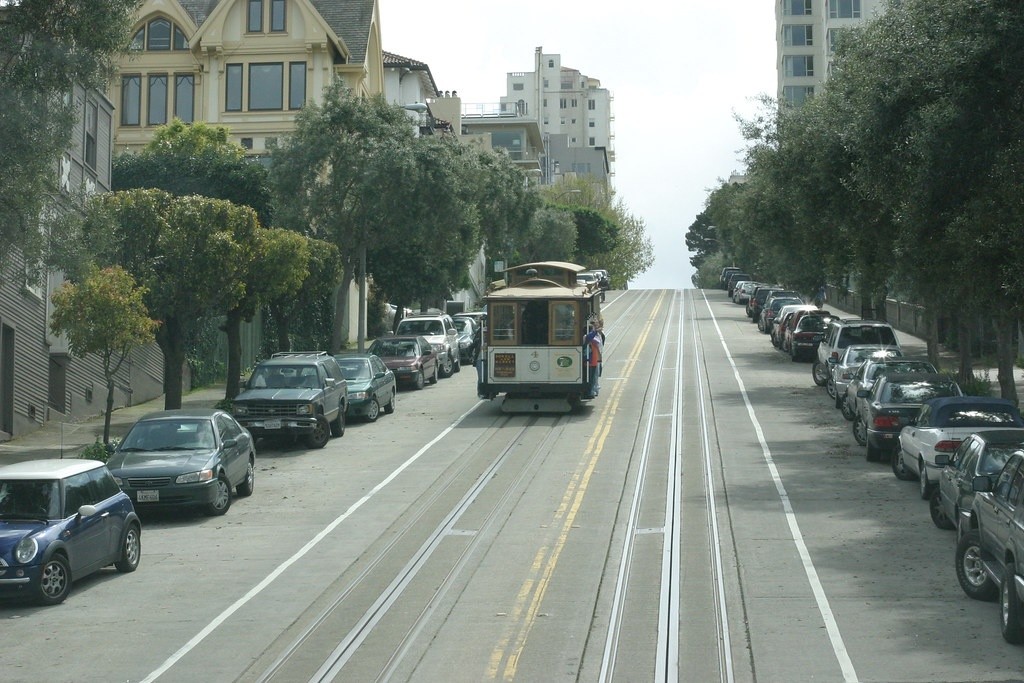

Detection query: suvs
[
  {"left": 812, "top": 318, "right": 901, "bottom": 387},
  {"left": 231, "top": 351, "right": 348, "bottom": 448},
  {"left": 386, "top": 312, "right": 460, "bottom": 378}
]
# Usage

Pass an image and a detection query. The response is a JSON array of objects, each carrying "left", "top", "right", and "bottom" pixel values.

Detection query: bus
[{"left": 473, "top": 261, "right": 605, "bottom": 413}]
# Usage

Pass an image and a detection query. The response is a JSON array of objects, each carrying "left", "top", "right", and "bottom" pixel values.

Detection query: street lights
[{"left": 357, "top": 103, "right": 428, "bottom": 351}]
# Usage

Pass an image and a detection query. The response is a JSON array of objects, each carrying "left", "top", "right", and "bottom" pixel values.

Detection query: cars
[
  {"left": 333, "top": 354, "right": 396, "bottom": 421},
  {"left": 366, "top": 335, "right": 439, "bottom": 389},
  {"left": 105, "top": 409, "right": 255, "bottom": 525},
  {"left": 0, "top": 459, "right": 142, "bottom": 605},
  {"left": 826, "top": 344, "right": 1024, "bottom": 544},
  {"left": 451, "top": 312, "right": 487, "bottom": 364},
  {"left": 956, "top": 443, "right": 1024, "bottom": 642},
  {"left": 719, "top": 267, "right": 840, "bottom": 361}
]
